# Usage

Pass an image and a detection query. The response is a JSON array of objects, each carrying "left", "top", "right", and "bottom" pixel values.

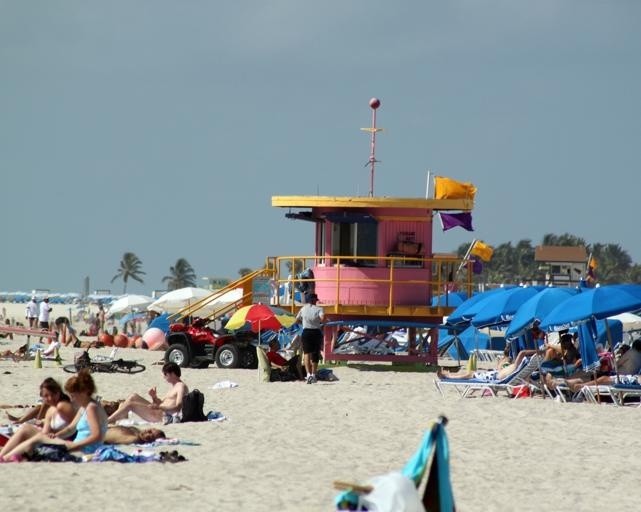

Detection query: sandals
[{"left": 158, "top": 450, "right": 187, "bottom": 464}]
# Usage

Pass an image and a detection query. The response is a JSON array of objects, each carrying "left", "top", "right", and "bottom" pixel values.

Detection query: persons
[
  {"left": 1, "top": 294, "right": 106, "bottom": 361},
  {"left": 295, "top": 292, "right": 329, "bottom": 383},
  {"left": 266, "top": 338, "right": 306, "bottom": 381},
  {"left": 1, "top": 362, "right": 189, "bottom": 463}
]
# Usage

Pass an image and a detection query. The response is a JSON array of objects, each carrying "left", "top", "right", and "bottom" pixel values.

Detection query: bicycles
[{"left": 62, "top": 354, "right": 145, "bottom": 375}]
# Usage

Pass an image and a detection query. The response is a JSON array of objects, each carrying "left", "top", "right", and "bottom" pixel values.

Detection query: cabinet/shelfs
[{"left": 385, "top": 251, "right": 425, "bottom": 269}]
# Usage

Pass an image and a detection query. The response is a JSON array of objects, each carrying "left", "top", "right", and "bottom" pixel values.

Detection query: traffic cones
[
  {"left": 464, "top": 353, "right": 477, "bottom": 371},
  {"left": 33, "top": 348, "right": 43, "bottom": 368},
  {"left": 52, "top": 346, "right": 62, "bottom": 365}
]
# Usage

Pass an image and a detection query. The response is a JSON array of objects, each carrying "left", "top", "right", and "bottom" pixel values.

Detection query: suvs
[{"left": 166, "top": 317, "right": 259, "bottom": 368}]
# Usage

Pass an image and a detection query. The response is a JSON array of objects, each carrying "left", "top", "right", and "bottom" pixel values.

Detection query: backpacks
[{"left": 178, "top": 387, "right": 208, "bottom": 423}]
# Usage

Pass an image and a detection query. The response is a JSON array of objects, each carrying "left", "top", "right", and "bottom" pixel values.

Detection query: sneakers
[{"left": 307, "top": 372, "right": 319, "bottom": 383}]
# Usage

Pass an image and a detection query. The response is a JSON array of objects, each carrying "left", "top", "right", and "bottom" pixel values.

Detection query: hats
[{"left": 305, "top": 293, "right": 319, "bottom": 302}]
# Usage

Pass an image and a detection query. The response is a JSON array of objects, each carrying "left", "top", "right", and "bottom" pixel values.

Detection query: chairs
[
  {"left": 213, "top": 317, "right": 231, "bottom": 337},
  {"left": 433, "top": 342, "right": 641, "bottom": 406},
  {"left": 332, "top": 415, "right": 447, "bottom": 512}
]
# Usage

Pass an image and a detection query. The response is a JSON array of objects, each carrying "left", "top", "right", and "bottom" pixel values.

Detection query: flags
[
  {"left": 463, "top": 255, "right": 483, "bottom": 275},
  {"left": 439, "top": 211, "right": 474, "bottom": 232},
  {"left": 470, "top": 241, "right": 495, "bottom": 263},
  {"left": 433, "top": 174, "right": 477, "bottom": 200}
]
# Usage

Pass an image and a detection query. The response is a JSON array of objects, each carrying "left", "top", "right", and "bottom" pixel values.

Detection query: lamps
[{"left": 285, "top": 207, "right": 376, "bottom": 226}]
